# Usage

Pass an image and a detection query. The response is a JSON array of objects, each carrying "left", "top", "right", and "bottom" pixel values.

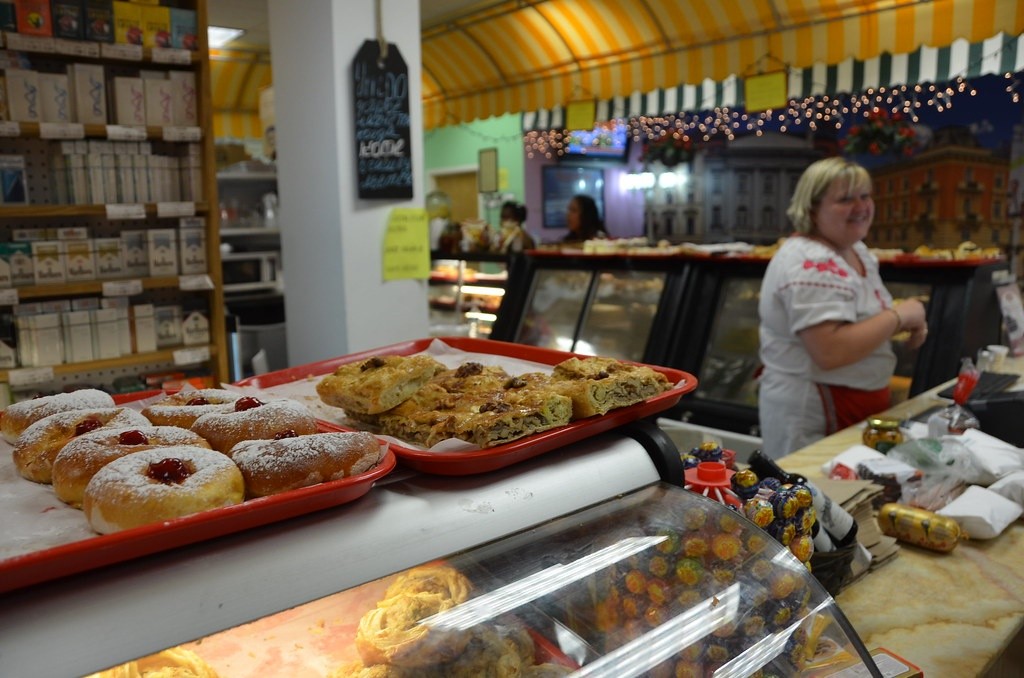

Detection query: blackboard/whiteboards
[{"left": 352, "top": 40, "right": 414, "bottom": 199}]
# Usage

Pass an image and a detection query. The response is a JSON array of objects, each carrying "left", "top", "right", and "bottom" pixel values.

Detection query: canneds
[{"left": 862, "top": 416, "right": 902, "bottom": 454}]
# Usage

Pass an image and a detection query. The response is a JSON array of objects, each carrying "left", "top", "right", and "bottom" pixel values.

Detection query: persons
[
  {"left": 759, "top": 158, "right": 927, "bottom": 460},
  {"left": 500, "top": 200, "right": 535, "bottom": 249},
  {"left": 563, "top": 196, "right": 607, "bottom": 241}
]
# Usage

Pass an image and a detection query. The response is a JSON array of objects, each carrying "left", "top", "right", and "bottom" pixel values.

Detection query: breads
[
  {"left": 3, "top": 388, "right": 382, "bottom": 536},
  {"left": 89, "top": 568, "right": 578, "bottom": 678}
]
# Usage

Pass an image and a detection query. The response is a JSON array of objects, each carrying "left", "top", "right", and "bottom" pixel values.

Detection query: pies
[{"left": 316, "top": 351, "right": 670, "bottom": 446}]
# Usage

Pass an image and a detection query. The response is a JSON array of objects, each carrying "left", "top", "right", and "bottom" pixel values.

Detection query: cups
[{"left": 986, "top": 346, "right": 1010, "bottom": 373}]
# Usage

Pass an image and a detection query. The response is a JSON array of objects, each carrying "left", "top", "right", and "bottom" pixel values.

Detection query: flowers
[
  {"left": 634, "top": 128, "right": 693, "bottom": 168},
  {"left": 837, "top": 105, "right": 925, "bottom": 162}
]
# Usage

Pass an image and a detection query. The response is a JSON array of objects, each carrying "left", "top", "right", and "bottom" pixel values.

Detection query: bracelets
[{"left": 887, "top": 307, "right": 901, "bottom": 335}]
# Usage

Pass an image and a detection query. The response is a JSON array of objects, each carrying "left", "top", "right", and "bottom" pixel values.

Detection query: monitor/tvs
[{"left": 563, "top": 118, "right": 633, "bottom": 163}]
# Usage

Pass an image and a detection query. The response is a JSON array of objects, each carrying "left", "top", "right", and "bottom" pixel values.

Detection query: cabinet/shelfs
[
  {"left": 218, "top": 169, "right": 281, "bottom": 293},
  {"left": 0, "top": 1, "right": 228, "bottom": 397}
]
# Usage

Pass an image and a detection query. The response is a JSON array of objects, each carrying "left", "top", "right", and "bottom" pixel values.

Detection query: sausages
[{"left": 873, "top": 503, "right": 969, "bottom": 552}]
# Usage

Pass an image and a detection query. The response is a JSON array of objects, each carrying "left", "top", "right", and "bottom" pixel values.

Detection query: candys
[{"left": 573, "top": 441, "right": 817, "bottom": 678}]
